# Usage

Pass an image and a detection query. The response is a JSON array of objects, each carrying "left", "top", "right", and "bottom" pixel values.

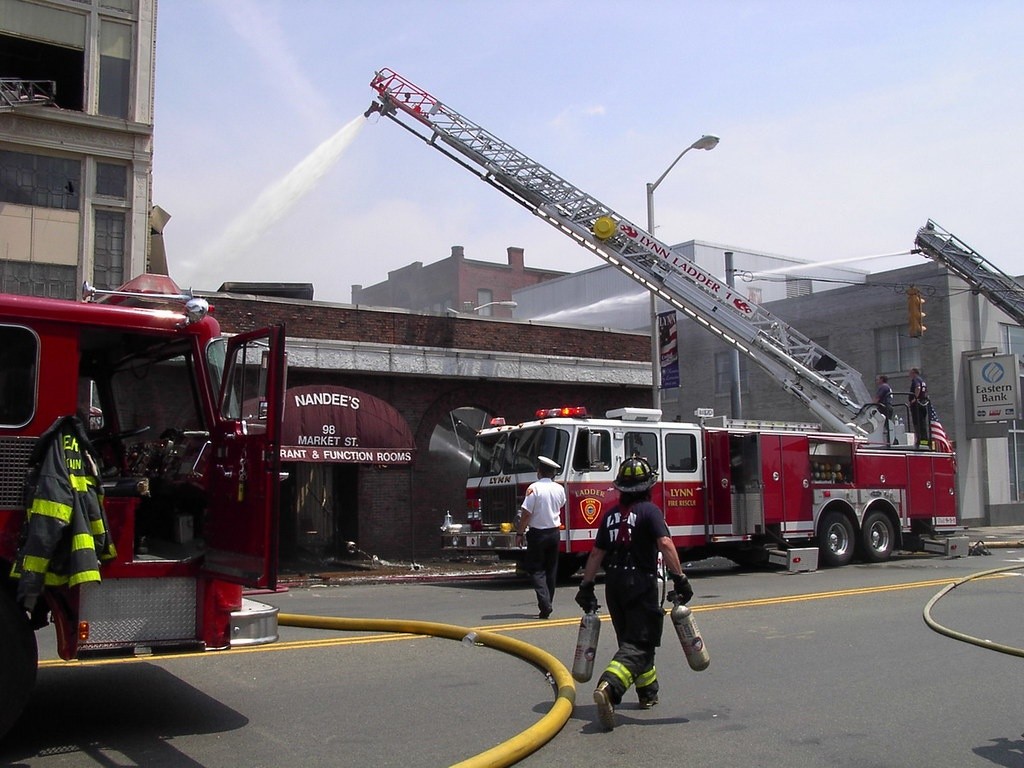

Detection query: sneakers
[
  {"left": 593, "top": 681, "right": 615, "bottom": 731},
  {"left": 640, "top": 697, "right": 659, "bottom": 709}
]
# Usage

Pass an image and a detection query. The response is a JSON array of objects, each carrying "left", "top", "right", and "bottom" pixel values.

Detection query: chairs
[{"left": 99, "top": 476, "right": 143, "bottom": 500}]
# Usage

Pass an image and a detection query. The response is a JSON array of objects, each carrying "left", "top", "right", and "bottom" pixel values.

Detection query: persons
[
  {"left": 876, "top": 374, "right": 893, "bottom": 446},
  {"left": 516, "top": 456, "right": 566, "bottom": 619},
  {"left": 908, "top": 367, "right": 928, "bottom": 447},
  {"left": 575, "top": 458, "right": 694, "bottom": 730}
]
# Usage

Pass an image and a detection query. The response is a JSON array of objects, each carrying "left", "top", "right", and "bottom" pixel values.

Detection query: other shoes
[{"left": 538, "top": 606, "right": 553, "bottom": 619}]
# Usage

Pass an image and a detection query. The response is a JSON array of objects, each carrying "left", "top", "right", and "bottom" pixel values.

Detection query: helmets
[{"left": 612, "top": 457, "right": 659, "bottom": 492}]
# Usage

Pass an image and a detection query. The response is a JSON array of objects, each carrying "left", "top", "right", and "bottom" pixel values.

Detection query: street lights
[{"left": 645, "top": 135, "right": 721, "bottom": 426}]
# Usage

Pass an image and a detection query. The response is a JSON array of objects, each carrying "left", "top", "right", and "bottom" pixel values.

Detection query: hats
[{"left": 538, "top": 455, "right": 561, "bottom": 469}]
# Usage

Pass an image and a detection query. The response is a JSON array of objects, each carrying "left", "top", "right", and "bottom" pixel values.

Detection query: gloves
[
  {"left": 673, "top": 573, "right": 694, "bottom": 605},
  {"left": 575, "top": 580, "right": 597, "bottom": 613}
]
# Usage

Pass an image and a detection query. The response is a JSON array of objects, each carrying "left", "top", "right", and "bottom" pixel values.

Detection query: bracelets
[{"left": 516, "top": 533, "right": 523, "bottom": 536}]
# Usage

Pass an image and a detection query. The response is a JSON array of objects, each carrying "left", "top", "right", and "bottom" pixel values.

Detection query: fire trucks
[
  {"left": 1, "top": 274, "right": 285, "bottom": 666},
  {"left": 365, "top": 61, "right": 962, "bottom": 568}
]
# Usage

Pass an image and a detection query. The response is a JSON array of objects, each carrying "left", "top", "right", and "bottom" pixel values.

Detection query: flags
[{"left": 930, "top": 404, "right": 952, "bottom": 453}]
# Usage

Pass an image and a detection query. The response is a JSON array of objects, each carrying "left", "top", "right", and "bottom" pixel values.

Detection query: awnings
[{"left": 235, "top": 385, "right": 418, "bottom": 465}]
